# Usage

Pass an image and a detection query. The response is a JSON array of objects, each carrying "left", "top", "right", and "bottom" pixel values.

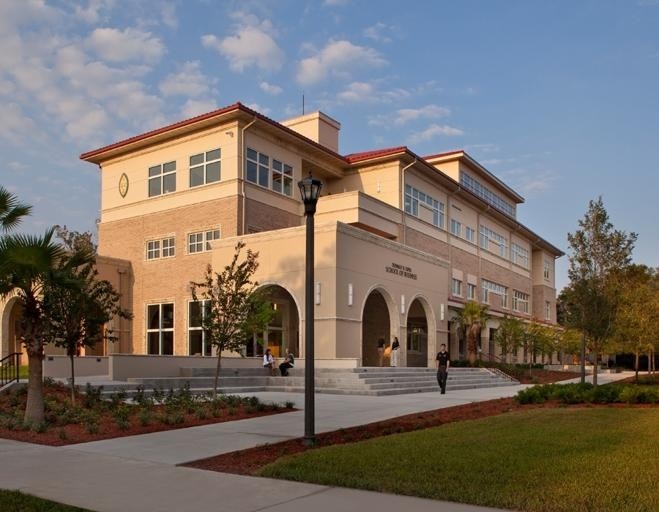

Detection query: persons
[
  {"left": 377, "top": 335, "right": 386, "bottom": 367},
  {"left": 279, "top": 348, "right": 294, "bottom": 376},
  {"left": 263, "top": 349, "right": 280, "bottom": 375},
  {"left": 436, "top": 344, "right": 450, "bottom": 394},
  {"left": 389, "top": 337, "right": 400, "bottom": 367}
]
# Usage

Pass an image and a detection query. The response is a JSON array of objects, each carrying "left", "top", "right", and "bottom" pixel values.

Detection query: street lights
[{"left": 298, "top": 170, "right": 322, "bottom": 451}]
